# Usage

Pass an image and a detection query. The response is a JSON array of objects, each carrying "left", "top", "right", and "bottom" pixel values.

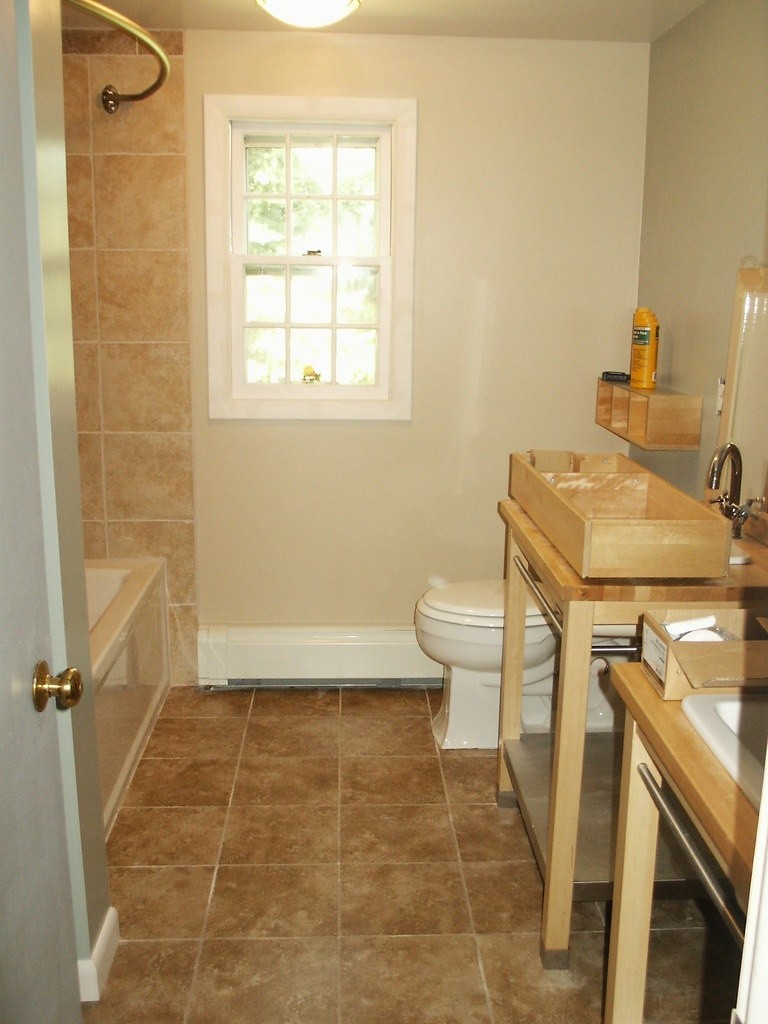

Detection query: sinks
[{"left": 683, "top": 692, "right": 765, "bottom": 812}]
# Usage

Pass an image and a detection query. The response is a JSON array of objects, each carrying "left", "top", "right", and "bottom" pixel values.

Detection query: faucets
[{"left": 706, "top": 444, "right": 759, "bottom": 540}]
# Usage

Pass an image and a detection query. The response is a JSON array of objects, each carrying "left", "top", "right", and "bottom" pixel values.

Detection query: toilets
[{"left": 413, "top": 577, "right": 626, "bottom": 749}]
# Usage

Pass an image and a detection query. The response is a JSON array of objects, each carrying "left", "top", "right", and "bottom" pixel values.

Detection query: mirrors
[{"left": 719, "top": 256, "right": 768, "bottom": 547}]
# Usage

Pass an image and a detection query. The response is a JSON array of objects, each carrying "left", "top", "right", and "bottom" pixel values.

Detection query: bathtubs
[{"left": 84, "top": 557, "right": 170, "bottom": 835}]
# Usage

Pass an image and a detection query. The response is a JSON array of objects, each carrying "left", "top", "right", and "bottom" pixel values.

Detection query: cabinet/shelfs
[{"left": 595, "top": 377, "right": 702, "bottom": 453}]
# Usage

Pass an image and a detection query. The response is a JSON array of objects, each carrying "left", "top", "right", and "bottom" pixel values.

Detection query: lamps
[{"left": 258, "top": 0, "right": 361, "bottom": 29}]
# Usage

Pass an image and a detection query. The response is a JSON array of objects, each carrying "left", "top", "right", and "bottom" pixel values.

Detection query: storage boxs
[{"left": 643, "top": 607, "right": 768, "bottom": 702}]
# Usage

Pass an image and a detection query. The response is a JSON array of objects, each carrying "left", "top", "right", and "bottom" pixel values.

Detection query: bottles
[{"left": 629, "top": 307, "right": 659, "bottom": 389}]
